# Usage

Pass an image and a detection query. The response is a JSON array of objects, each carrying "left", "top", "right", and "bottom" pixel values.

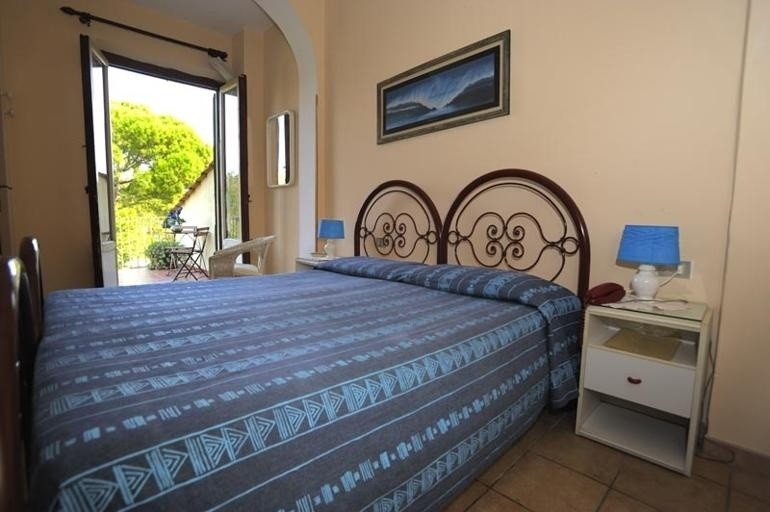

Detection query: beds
[{"left": 2, "top": 164, "right": 598, "bottom": 509}]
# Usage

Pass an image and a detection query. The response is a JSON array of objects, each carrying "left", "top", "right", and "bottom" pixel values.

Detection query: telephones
[{"left": 585, "top": 283, "right": 625, "bottom": 305}]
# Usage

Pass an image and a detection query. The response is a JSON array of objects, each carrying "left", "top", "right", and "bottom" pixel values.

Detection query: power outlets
[{"left": 671, "top": 259, "right": 692, "bottom": 281}]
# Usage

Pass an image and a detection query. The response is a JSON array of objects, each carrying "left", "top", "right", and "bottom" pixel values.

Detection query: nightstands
[
  {"left": 293, "top": 254, "right": 341, "bottom": 272},
  {"left": 570, "top": 292, "right": 718, "bottom": 477}
]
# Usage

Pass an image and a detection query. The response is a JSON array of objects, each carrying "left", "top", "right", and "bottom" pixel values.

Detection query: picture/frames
[{"left": 375, "top": 27, "right": 513, "bottom": 150}]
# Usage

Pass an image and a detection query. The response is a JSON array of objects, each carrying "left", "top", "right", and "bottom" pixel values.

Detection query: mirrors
[{"left": 263, "top": 110, "right": 296, "bottom": 189}]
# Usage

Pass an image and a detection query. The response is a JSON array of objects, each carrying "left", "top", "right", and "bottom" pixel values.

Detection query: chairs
[
  {"left": 207, "top": 234, "right": 276, "bottom": 280},
  {"left": 163, "top": 222, "right": 211, "bottom": 281}
]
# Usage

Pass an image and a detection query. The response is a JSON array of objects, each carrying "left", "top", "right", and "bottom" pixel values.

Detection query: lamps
[
  {"left": 614, "top": 220, "right": 682, "bottom": 304},
  {"left": 316, "top": 218, "right": 347, "bottom": 260}
]
